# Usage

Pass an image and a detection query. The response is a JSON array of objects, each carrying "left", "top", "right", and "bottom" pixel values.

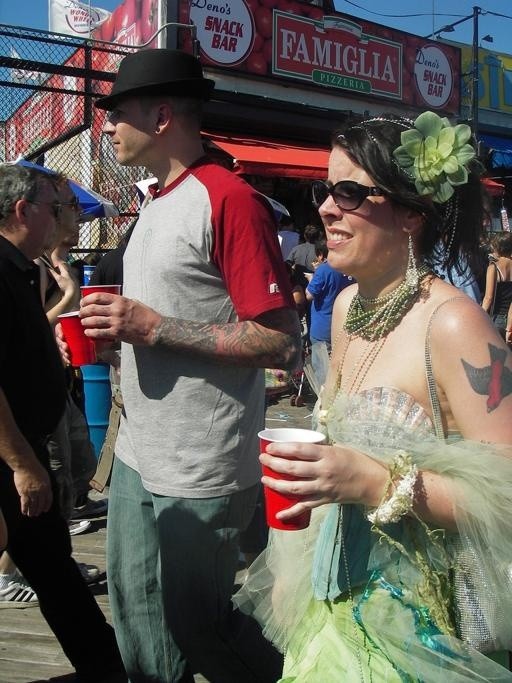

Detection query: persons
[
  {"left": 229, "top": 110, "right": 512, "bottom": 683},
  {"left": 422, "top": 226, "right": 512, "bottom": 351},
  {"left": 55, "top": 48, "right": 305, "bottom": 682},
  {"left": 276, "top": 222, "right": 355, "bottom": 409},
  {"left": 1, "top": 162, "right": 129, "bottom": 683}
]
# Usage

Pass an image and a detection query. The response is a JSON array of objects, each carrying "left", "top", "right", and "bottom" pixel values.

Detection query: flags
[
  {"left": 11, "top": 47, "right": 47, "bottom": 80},
  {"left": 48, "top": 0, "right": 114, "bottom": 40}
]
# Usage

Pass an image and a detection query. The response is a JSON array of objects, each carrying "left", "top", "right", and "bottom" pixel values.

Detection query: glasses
[
  {"left": 58, "top": 197, "right": 77, "bottom": 208},
  {"left": 312, "top": 180, "right": 387, "bottom": 212},
  {"left": 26, "top": 199, "right": 59, "bottom": 217}
]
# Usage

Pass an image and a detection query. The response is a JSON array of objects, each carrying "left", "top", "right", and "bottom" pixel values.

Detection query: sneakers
[
  {"left": 77, "top": 562, "right": 98, "bottom": 582},
  {"left": 68, "top": 521, "right": 91, "bottom": 536},
  {"left": 71, "top": 498, "right": 108, "bottom": 518},
  {"left": 0, "top": 575, "right": 39, "bottom": 608}
]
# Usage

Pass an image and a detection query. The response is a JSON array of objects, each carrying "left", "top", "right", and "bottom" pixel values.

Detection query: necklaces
[{"left": 327, "top": 262, "right": 432, "bottom": 415}]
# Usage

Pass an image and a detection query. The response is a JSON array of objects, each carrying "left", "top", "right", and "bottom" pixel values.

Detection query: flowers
[{"left": 393, "top": 111, "right": 476, "bottom": 204}]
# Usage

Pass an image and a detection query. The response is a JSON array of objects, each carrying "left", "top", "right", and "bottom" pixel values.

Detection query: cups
[
  {"left": 56, "top": 310, "right": 98, "bottom": 370},
  {"left": 83, "top": 265, "right": 96, "bottom": 285},
  {"left": 77, "top": 284, "right": 123, "bottom": 342},
  {"left": 256, "top": 425, "right": 327, "bottom": 533}
]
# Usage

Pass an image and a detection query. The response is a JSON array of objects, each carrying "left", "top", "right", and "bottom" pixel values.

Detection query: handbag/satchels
[{"left": 447, "top": 533, "right": 512, "bottom": 656}]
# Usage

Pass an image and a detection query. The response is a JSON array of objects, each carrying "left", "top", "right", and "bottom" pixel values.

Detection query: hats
[{"left": 95, "top": 50, "right": 216, "bottom": 111}]
